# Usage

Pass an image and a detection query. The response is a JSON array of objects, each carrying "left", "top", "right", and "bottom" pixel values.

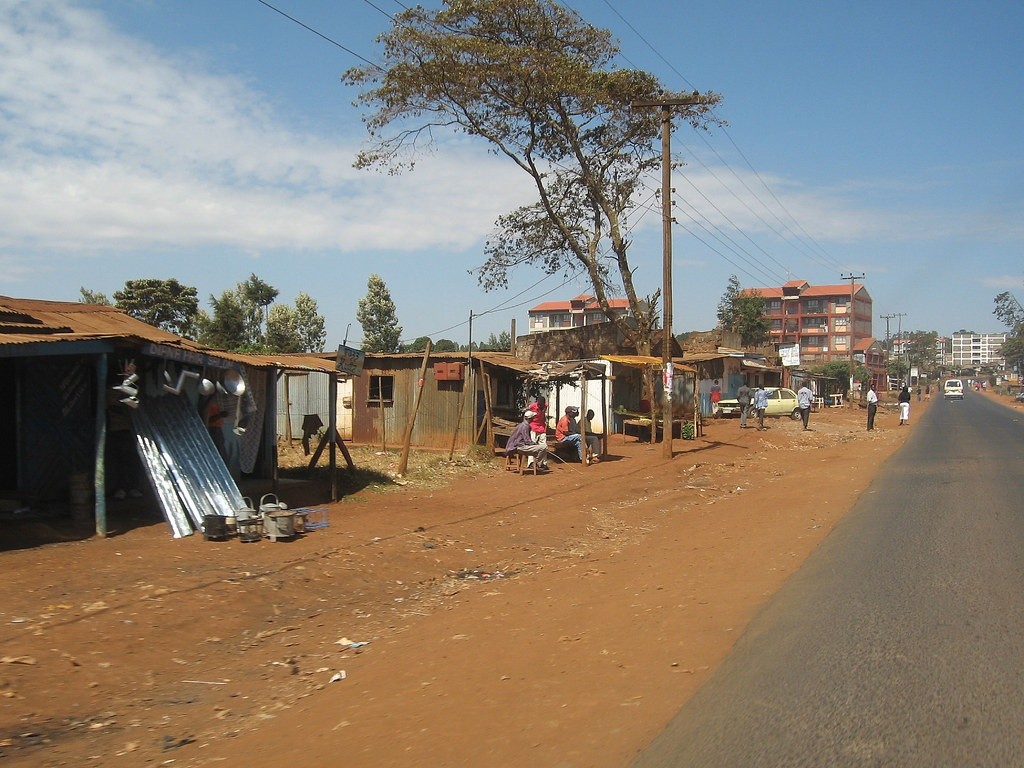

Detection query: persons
[
  {"left": 967, "top": 379, "right": 987, "bottom": 392},
  {"left": 797, "top": 382, "right": 813, "bottom": 431},
  {"left": 936, "top": 377, "right": 941, "bottom": 391},
  {"left": 866, "top": 384, "right": 878, "bottom": 432},
  {"left": 556, "top": 406, "right": 593, "bottom": 465},
  {"left": 505, "top": 396, "right": 552, "bottom": 474},
  {"left": 1007, "top": 386, "right": 1010, "bottom": 394},
  {"left": 737, "top": 382, "right": 753, "bottom": 429},
  {"left": 916, "top": 384, "right": 932, "bottom": 402},
  {"left": 710, "top": 380, "right": 721, "bottom": 419},
  {"left": 751, "top": 384, "right": 773, "bottom": 431},
  {"left": 107, "top": 380, "right": 145, "bottom": 502},
  {"left": 203, "top": 393, "right": 230, "bottom": 469},
  {"left": 897, "top": 385, "right": 910, "bottom": 426},
  {"left": 576, "top": 409, "right": 601, "bottom": 463}
]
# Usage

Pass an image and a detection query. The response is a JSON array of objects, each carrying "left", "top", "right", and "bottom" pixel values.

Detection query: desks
[{"left": 623, "top": 420, "right": 684, "bottom": 444}]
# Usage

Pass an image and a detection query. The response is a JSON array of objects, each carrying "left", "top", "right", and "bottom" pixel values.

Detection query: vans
[{"left": 943, "top": 380, "right": 964, "bottom": 400}]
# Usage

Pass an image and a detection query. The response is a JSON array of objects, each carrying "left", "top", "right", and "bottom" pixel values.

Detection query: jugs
[{"left": 233, "top": 493, "right": 287, "bottom": 533}]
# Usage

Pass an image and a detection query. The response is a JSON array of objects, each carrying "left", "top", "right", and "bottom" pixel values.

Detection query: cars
[{"left": 715, "top": 387, "right": 802, "bottom": 423}]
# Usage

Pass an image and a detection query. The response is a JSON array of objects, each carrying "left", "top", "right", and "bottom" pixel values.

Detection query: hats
[
  {"left": 525, "top": 410, "right": 537, "bottom": 419},
  {"left": 571, "top": 406, "right": 580, "bottom": 411},
  {"left": 565, "top": 405, "right": 573, "bottom": 413},
  {"left": 538, "top": 396, "right": 546, "bottom": 409}
]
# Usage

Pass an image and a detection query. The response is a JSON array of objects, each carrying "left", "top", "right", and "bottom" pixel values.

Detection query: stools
[
  {"left": 505, "top": 454, "right": 520, "bottom": 472},
  {"left": 521, "top": 453, "right": 536, "bottom": 476}
]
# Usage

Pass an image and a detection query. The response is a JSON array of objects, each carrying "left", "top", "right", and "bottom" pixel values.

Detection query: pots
[{"left": 198, "top": 353, "right": 247, "bottom": 398}]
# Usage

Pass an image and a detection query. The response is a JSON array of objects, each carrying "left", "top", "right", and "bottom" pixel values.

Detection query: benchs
[{"left": 552, "top": 442, "right": 590, "bottom": 463}]
